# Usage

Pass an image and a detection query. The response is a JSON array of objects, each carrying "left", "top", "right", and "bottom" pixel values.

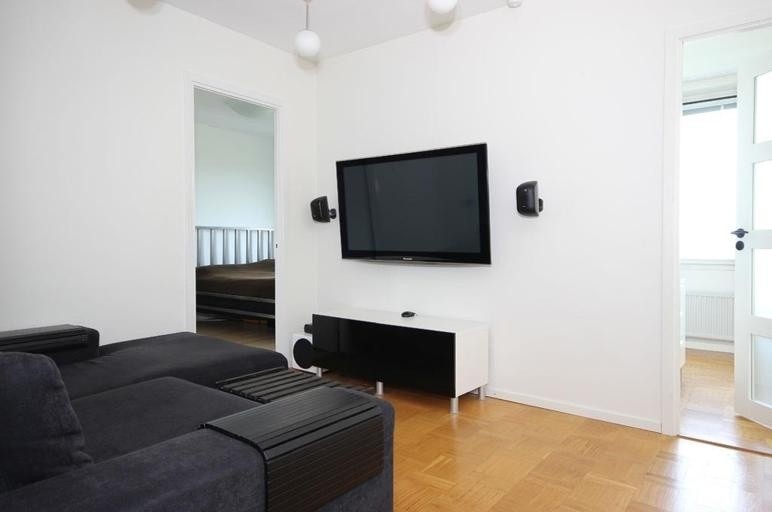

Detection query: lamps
[{"left": 293, "top": 1, "right": 320, "bottom": 61}]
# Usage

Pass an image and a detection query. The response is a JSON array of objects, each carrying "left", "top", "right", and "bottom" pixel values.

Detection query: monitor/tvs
[{"left": 336, "top": 143, "right": 491, "bottom": 265}]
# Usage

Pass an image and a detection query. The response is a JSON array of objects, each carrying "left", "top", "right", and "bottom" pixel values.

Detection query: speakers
[
  {"left": 311, "top": 195, "right": 330, "bottom": 223},
  {"left": 516, "top": 180, "right": 539, "bottom": 216}
]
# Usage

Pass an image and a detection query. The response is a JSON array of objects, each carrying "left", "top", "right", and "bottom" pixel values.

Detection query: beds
[{"left": 196, "top": 225, "right": 274, "bottom": 327}]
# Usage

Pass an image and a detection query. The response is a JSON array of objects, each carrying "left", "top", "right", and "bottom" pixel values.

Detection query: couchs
[{"left": 1, "top": 324, "right": 395, "bottom": 511}]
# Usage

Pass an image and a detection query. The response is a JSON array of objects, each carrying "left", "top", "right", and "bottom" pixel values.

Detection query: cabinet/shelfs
[{"left": 312, "top": 315, "right": 489, "bottom": 415}]
